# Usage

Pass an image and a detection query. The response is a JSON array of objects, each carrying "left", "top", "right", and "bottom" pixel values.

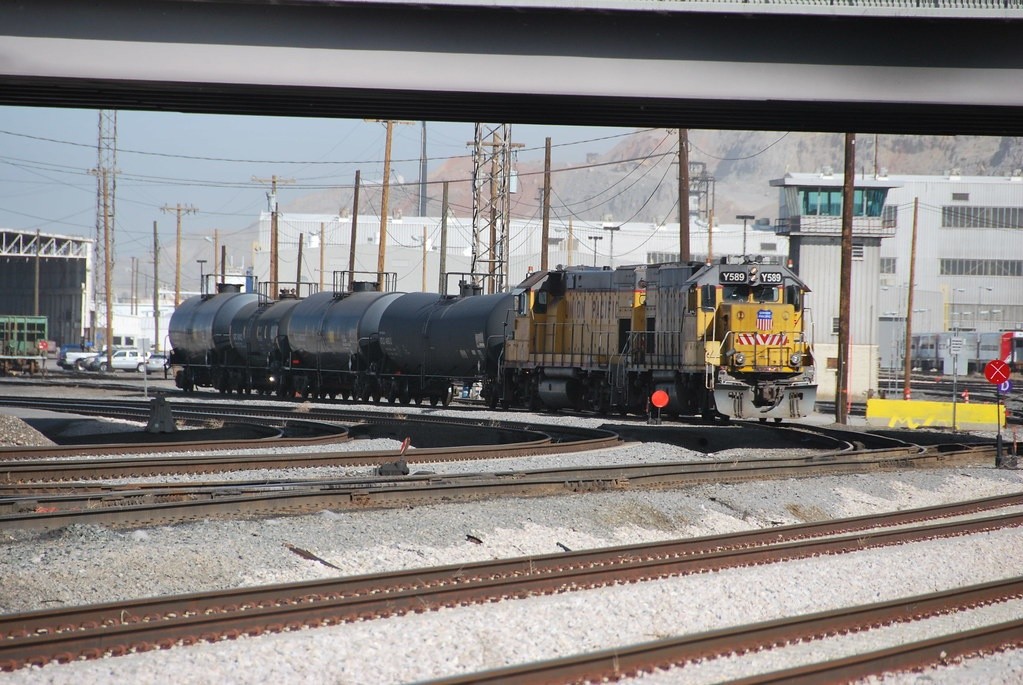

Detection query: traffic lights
[{"left": 649, "top": 389, "right": 669, "bottom": 410}]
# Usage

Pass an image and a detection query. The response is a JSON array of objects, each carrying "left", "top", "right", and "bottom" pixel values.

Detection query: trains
[
  {"left": 166, "top": 258, "right": 818, "bottom": 425},
  {"left": 902, "top": 330, "right": 1023, "bottom": 372}
]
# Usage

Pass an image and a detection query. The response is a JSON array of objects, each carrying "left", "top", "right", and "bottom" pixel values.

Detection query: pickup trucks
[{"left": 57, "top": 343, "right": 125, "bottom": 372}]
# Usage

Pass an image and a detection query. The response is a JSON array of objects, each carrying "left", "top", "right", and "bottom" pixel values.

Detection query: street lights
[
  {"left": 603, "top": 227, "right": 620, "bottom": 267},
  {"left": 977, "top": 286, "right": 992, "bottom": 332},
  {"left": 952, "top": 287, "right": 963, "bottom": 332},
  {"left": 735, "top": 215, "right": 755, "bottom": 255},
  {"left": 588, "top": 236, "right": 602, "bottom": 266}
]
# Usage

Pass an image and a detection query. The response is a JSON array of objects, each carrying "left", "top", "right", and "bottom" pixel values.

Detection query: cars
[
  {"left": 80, "top": 348, "right": 150, "bottom": 372},
  {"left": 145, "top": 354, "right": 171, "bottom": 375}
]
